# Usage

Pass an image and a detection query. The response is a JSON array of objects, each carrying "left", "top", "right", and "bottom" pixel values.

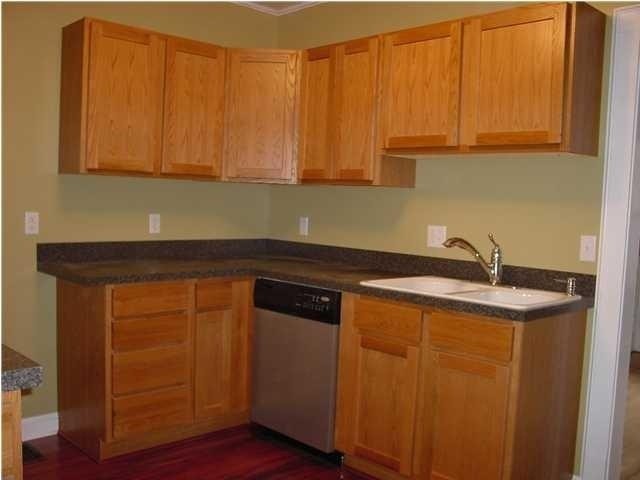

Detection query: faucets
[{"left": 442, "top": 232, "right": 505, "bottom": 285}]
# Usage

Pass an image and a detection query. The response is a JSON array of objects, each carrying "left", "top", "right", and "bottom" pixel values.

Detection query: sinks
[
  {"left": 449, "top": 286, "right": 566, "bottom": 307},
  {"left": 366, "top": 273, "right": 485, "bottom": 295}
]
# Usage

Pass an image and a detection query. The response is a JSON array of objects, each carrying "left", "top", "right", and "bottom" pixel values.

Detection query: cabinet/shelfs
[
  {"left": 59, "top": 16, "right": 228, "bottom": 182},
  {"left": 299, "top": 35, "right": 416, "bottom": 187},
  {"left": 222, "top": 48, "right": 301, "bottom": 184},
  {"left": 333, "top": 292, "right": 587, "bottom": 480},
  {"left": 381, "top": 1, "right": 606, "bottom": 158},
  {"left": 56, "top": 275, "right": 252, "bottom": 465}
]
